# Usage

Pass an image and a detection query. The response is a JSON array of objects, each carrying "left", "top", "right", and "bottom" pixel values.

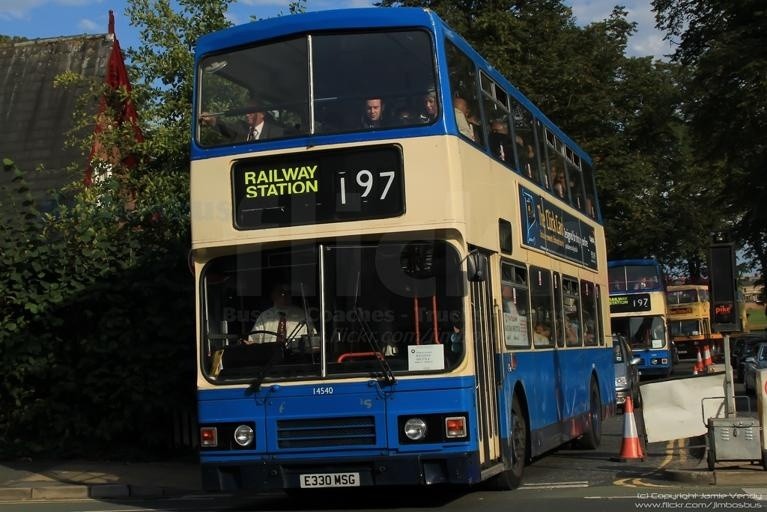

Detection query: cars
[
  {"left": 613, "top": 335, "right": 641, "bottom": 412},
  {"left": 732, "top": 334, "right": 766, "bottom": 469}
]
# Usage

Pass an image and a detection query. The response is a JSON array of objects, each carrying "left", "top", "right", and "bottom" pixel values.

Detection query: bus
[
  {"left": 667, "top": 285, "right": 766, "bottom": 357},
  {"left": 608, "top": 260, "right": 674, "bottom": 378},
  {"left": 189, "top": 6, "right": 618, "bottom": 491}
]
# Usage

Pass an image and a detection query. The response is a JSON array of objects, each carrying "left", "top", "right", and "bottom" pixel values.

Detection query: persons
[
  {"left": 395, "top": 87, "right": 598, "bottom": 218},
  {"left": 350, "top": 96, "right": 413, "bottom": 128},
  {"left": 199, "top": 98, "right": 295, "bottom": 139},
  {"left": 236, "top": 280, "right": 317, "bottom": 345},
  {"left": 608, "top": 272, "right": 661, "bottom": 291},
  {"left": 450, "top": 317, "right": 603, "bottom": 347}
]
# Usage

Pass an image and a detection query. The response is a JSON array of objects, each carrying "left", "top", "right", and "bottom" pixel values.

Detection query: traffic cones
[
  {"left": 692, "top": 345, "right": 714, "bottom": 376},
  {"left": 611, "top": 396, "right": 646, "bottom": 463}
]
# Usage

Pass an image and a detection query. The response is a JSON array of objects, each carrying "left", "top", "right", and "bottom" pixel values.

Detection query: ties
[
  {"left": 277, "top": 312, "right": 286, "bottom": 347},
  {"left": 250, "top": 129, "right": 258, "bottom": 141}
]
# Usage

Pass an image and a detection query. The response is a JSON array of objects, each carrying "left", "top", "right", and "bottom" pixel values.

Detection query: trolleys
[{"left": 702, "top": 396, "right": 763, "bottom": 471}]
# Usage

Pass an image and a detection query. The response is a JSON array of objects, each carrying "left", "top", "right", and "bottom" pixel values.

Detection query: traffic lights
[{"left": 707, "top": 241, "right": 741, "bottom": 333}]
